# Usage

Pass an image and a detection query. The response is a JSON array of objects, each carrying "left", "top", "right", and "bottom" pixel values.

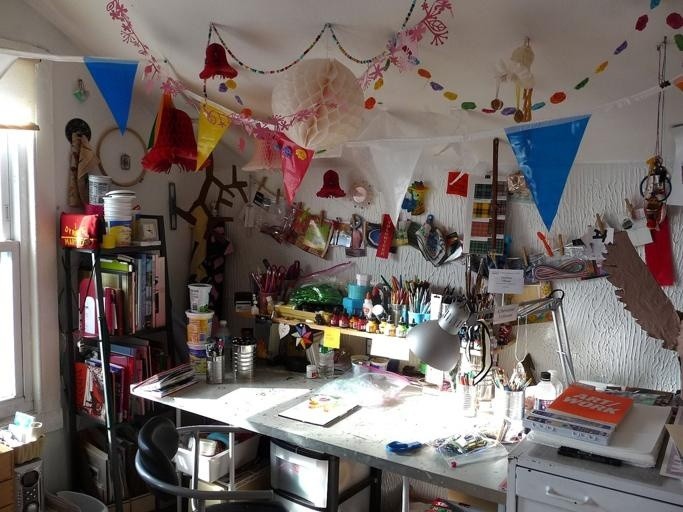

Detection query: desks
[{"left": 128, "top": 349, "right": 510, "bottom": 512}]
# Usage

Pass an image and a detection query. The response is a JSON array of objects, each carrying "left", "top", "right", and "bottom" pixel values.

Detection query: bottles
[
  {"left": 534, "top": 370, "right": 563, "bottom": 410},
  {"left": 216, "top": 320, "right": 231, "bottom": 369},
  {"left": 350, "top": 354, "right": 389, "bottom": 380},
  {"left": 307, "top": 365, "right": 316, "bottom": 378},
  {"left": 314, "top": 306, "right": 417, "bottom": 338},
  {"left": 31, "top": 422, "right": 42, "bottom": 440},
  {"left": 319, "top": 331, "right": 334, "bottom": 379},
  {"left": 252, "top": 294, "right": 260, "bottom": 315},
  {"left": 266, "top": 296, "right": 274, "bottom": 315}
]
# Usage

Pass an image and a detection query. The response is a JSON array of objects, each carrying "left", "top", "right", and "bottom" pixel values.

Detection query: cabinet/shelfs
[
  {"left": 58, "top": 214, "right": 179, "bottom": 512},
  {"left": 503, "top": 433, "right": 683, "bottom": 511},
  {"left": 267, "top": 435, "right": 382, "bottom": 512},
  {"left": 0, "top": 443, "right": 17, "bottom": 511}
]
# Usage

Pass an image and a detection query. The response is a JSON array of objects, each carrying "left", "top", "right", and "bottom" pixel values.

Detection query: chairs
[{"left": 132, "top": 414, "right": 287, "bottom": 511}]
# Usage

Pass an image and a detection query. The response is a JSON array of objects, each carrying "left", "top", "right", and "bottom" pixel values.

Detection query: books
[
  {"left": 278, "top": 394, "right": 358, "bottom": 425},
  {"left": 75, "top": 253, "right": 199, "bottom": 505},
  {"left": 546, "top": 382, "right": 636, "bottom": 429}
]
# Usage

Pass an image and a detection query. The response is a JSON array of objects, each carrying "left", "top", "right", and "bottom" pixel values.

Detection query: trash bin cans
[{"left": 57, "top": 492, "right": 107, "bottom": 511}]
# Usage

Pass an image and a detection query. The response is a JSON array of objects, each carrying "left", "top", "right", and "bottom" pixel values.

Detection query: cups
[
  {"left": 258, "top": 292, "right": 277, "bottom": 314},
  {"left": 233, "top": 336, "right": 258, "bottom": 379},
  {"left": 186, "top": 284, "right": 225, "bottom": 384},
  {"left": 89, "top": 175, "right": 136, "bottom": 248},
  {"left": 344, "top": 274, "right": 452, "bottom": 328},
  {"left": 459, "top": 349, "right": 525, "bottom": 419}
]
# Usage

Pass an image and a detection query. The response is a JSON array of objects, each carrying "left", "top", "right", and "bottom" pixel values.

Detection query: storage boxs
[{"left": 173, "top": 431, "right": 262, "bottom": 483}]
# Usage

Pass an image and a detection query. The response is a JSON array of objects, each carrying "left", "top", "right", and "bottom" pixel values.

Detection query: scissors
[{"left": 272, "top": 265, "right": 286, "bottom": 278}]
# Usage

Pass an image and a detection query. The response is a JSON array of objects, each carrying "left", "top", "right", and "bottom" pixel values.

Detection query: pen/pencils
[
  {"left": 218, "top": 339, "right": 223, "bottom": 356},
  {"left": 379, "top": 274, "right": 465, "bottom": 311},
  {"left": 248, "top": 270, "right": 280, "bottom": 293},
  {"left": 490, "top": 367, "right": 534, "bottom": 391},
  {"left": 457, "top": 370, "right": 475, "bottom": 384}
]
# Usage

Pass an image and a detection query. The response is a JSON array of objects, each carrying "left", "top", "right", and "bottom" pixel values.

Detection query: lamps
[{"left": 403, "top": 288, "right": 577, "bottom": 390}]
